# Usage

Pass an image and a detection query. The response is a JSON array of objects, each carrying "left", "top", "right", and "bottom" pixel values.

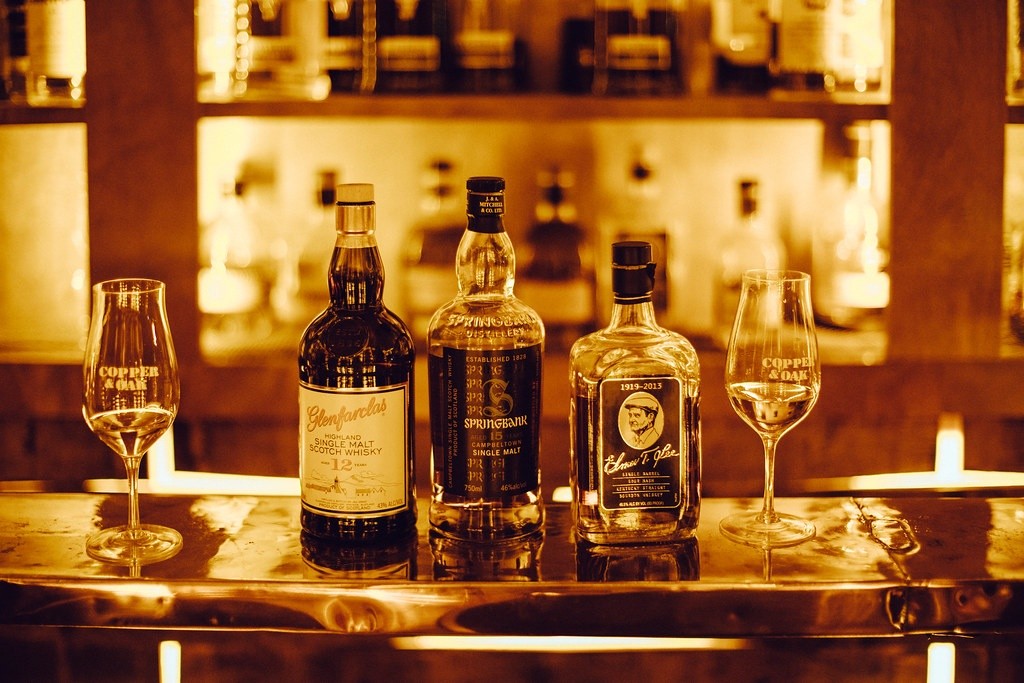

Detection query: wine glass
[
  {"left": 720, "top": 269, "right": 820, "bottom": 545},
  {"left": 82, "top": 278, "right": 182, "bottom": 562}
]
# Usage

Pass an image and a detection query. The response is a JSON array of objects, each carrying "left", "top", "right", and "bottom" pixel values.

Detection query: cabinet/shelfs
[{"left": 0, "top": 1, "right": 1023, "bottom": 497}]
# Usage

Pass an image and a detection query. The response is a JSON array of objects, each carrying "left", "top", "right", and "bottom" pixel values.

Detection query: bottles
[
  {"left": 203, "top": 181, "right": 276, "bottom": 334},
  {"left": 515, "top": 159, "right": 595, "bottom": 354},
  {"left": 428, "top": 177, "right": 544, "bottom": 542},
  {"left": 814, "top": 121, "right": 880, "bottom": 329},
  {"left": 290, "top": 169, "right": 337, "bottom": 335},
  {"left": 27, "top": 0, "right": 87, "bottom": 107},
  {"left": 298, "top": 183, "right": 417, "bottom": 541},
  {"left": 229, "top": 0, "right": 515, "bottom": 102},
  {"left": 613, "top": 144, "right": 674, "bottom": 325},
  {"left": 710, "top": 0, "right": 883, "bottom": 92},
  {"left": 556, "top": 0, "right": 688, "bottom": 97},
  {"left": 712, "top": 180, "right": 785, "bottom": 350},
  {"left": 569, "top": 241, "right": 701, "bottom": 545},
  {"left": 398, "top": 158, "right": 467, "bottom": 358}
]
